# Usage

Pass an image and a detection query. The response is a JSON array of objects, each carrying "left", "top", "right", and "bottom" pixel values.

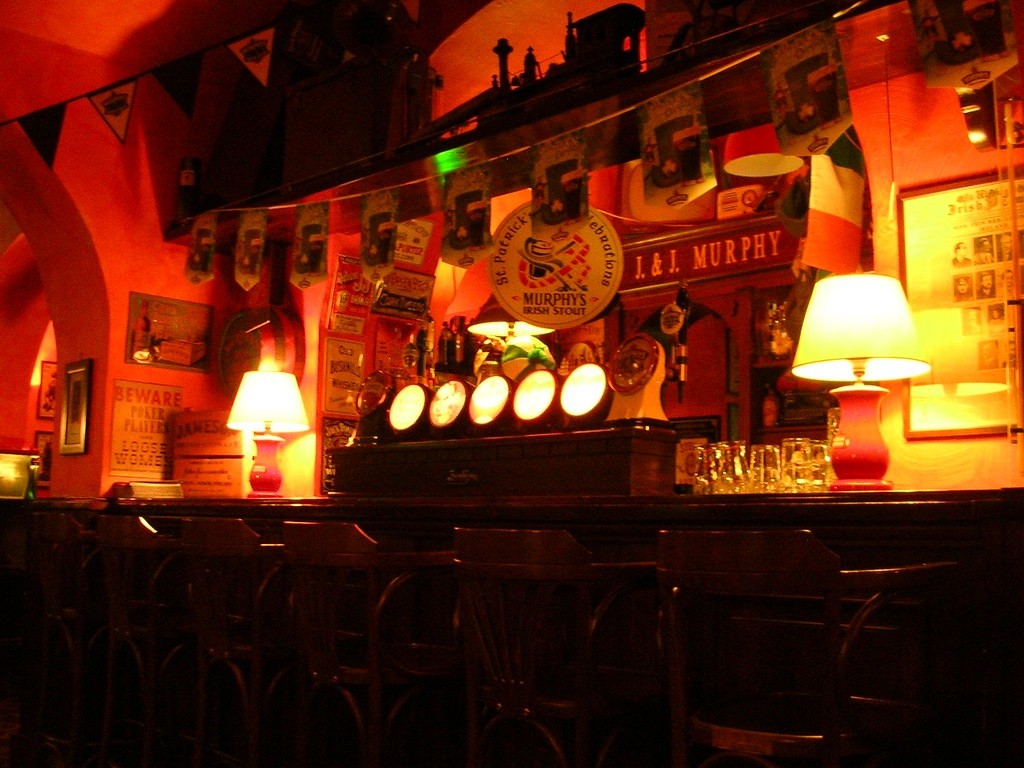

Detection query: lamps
[
  {"left": 227, "top": 371, "right": 311, "bottom": 498},
  {"left": 791, "top": 273, "right": 934, "bottom": 490},
  {"left": 722, "top": 123, "right": 805, "bottom": 177}
]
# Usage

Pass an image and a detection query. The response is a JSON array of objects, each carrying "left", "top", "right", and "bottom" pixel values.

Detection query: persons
[
  {"left": 975, "top": 239, "right": 993, "bottom": 265},
  {"left": 999, "top": 232, "right": 1013, "bottom": 260},
  {"left": 977, "top": 272, "right": 995, "bottom": 298},
  {"left": 983, "top": 344, "right": 998, "bottom": 369},
  {"left": 955, "top": 276, "right": 972, "bottom": 299},
  {"left": 952, "top": 243, "right": 972, "bottom": 270},
  {"left": 992, "top": 306, "right": 1001, "bottom": 320},
  {"left": 1001, "top": 270, "right": 1013, "bottom": 294}
]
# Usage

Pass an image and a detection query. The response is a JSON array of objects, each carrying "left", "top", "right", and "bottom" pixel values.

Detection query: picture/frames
[
  {"left": 126, "top": 290, "right": 215, "bottom": 373},
  {"left": 37, "top": 361, "right": 58, "bottom": 417},
  {"left": 34, "top": 430, "right": 54, "bottom": 489},
  {"left": 58, "top": 358, "right": 94, "bottom": 455}
]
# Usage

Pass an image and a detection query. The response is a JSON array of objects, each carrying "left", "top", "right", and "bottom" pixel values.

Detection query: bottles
[
  {"left": 438, "top": 321, "right": 456, "bottom": 372},
  {"left": 455, "top": 317, "right": 475, "bottom": 373},
  {"left": 769, "top": 313, "right": 793, "bottom": 360},
  {"left": 762, "top": 383, "right": 781, "bottom": 428},
  {"left": 25, "top": 455, "right": 41, "bottom": 501}
]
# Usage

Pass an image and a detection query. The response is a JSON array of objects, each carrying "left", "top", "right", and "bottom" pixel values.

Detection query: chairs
[{"left": 23, "top": 512, "right": 969, "bottom": 768}]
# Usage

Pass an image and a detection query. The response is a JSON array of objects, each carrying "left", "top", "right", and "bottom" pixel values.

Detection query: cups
[
  {"left": 693, "top": 437, "right": 838, "bottom": 497},
  {"left": 827, "top": 407, "right": 842, "bottom": 448}
]
